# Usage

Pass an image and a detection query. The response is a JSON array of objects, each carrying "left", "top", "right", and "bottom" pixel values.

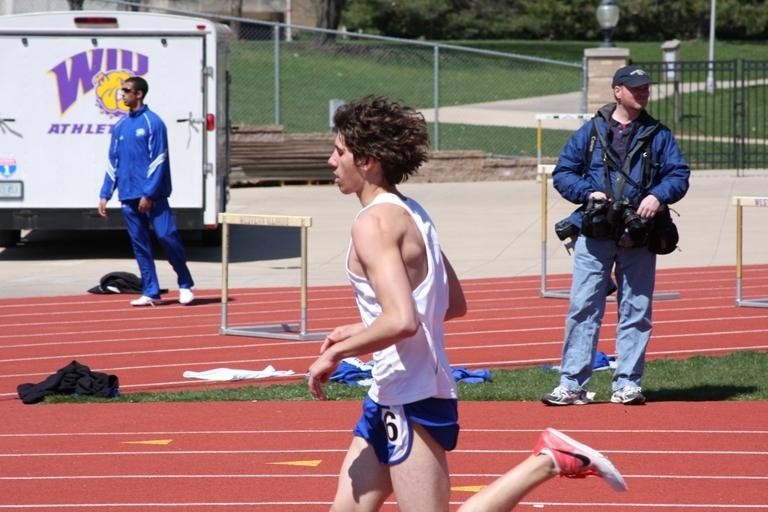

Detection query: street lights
[{"left": 596, "top": 1, "right": 621, "bottom": 48}]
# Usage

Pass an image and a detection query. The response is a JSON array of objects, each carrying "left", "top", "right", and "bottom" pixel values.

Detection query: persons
[
  {"left": 98, "top": 77, "right": 194, "bottom": 305},
  {"left": 308, "top": 92, "right": 629, "bottom": 510},
  {"left": 541, "top": 63, "right": 692, "bottom": 408}
]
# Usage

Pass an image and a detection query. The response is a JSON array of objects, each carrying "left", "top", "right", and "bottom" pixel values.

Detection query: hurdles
[
  {"left": 536, "top": 165, "right": 680, "bottom": 302},
  {"left": 216, "top": 213, "right": 333, "bottom": 340},
  {"left": 732, "top": 195, "right": 768, "bottom": 308}
]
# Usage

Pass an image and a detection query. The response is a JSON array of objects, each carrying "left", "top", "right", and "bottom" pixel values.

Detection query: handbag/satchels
[
  {"left": 650, "top": 204, "right": 679, "bottom": 256},
  {"left": 580, "top": 198, "right": 626, "bottom": 243}
]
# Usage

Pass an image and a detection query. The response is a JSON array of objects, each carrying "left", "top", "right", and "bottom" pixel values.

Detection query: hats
[{"left": 611, "top": 65, "right": 652, "bottom": 89}]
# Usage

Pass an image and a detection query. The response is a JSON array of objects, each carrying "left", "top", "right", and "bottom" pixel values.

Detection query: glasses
[{"left": 122, "top": 88, "right": 136, "bottom": 94}]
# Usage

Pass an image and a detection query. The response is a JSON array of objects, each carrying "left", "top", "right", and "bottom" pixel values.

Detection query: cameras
[{"left": 611, "top": 203, "right": 650, "bottom": 249}]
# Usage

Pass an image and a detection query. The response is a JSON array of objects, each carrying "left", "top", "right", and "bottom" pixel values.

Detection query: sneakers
[
  {"left": 531, "top": 427, "right": 627, "bottom": 492},
  {"left": 541, "top": 385, "right": 588, "bottom": 405},
  {"left": 610, "top": 385, "right": 646, "bottom": 406},
  {"left": 130, "top": 295, "right": 163, "bottom": 307},
  {"left": 177, "top": 287, "right": 195, "bottom": 305}
]
